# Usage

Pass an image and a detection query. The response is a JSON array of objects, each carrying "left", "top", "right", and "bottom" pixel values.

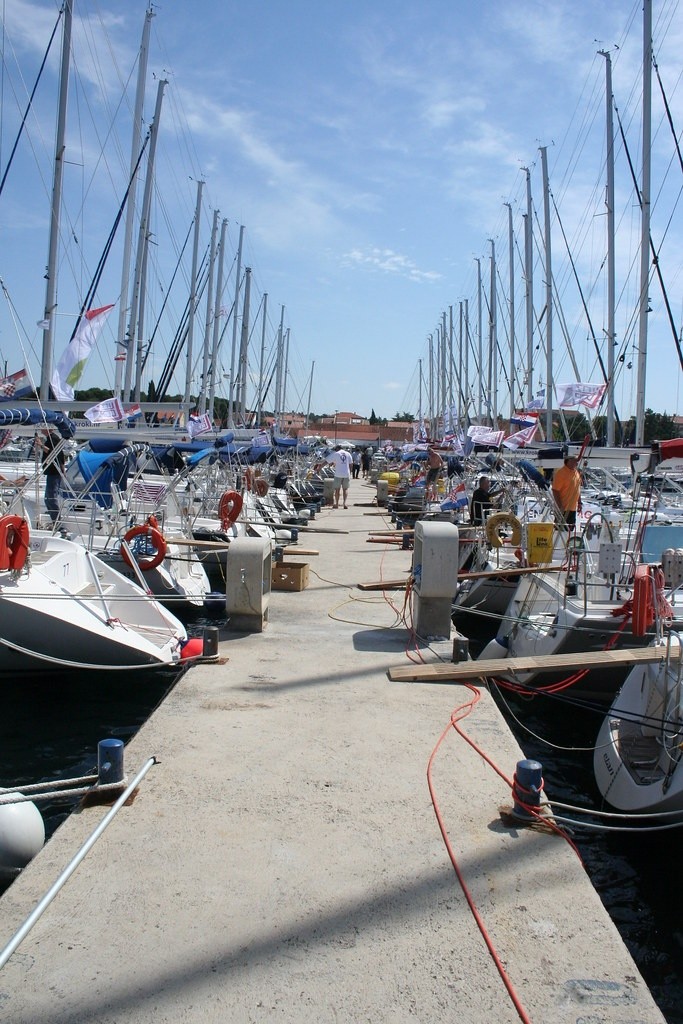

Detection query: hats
[
  {"left": 334, "top": 445, "right": 342, "bottom": 450},
  {"left": 565, "top": 455, "right": 576, "bottom": 460}
]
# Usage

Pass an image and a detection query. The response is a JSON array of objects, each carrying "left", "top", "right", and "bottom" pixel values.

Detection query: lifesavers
[
  {"left": 218, "top": 490, "right": 243, "bottom": 530},
  {"left": 486, "top": 511, "right": 523, "bottom": 548},
  {"left": 0, "top": 514, "right": 30, "bottom": 570},
  {"left": 120, "top": 525, "right": 166, "bottom": 571},
  {"left": 149, "top": 516, "right": 160, "bottom": 549},
  {"left": 243, "top": 467, "right": 252, "bottom": 491},
  {"left": 632, "top": 564, "right": 654, "bottom": 638},
  {"left": 252, "top": 480, "right": 269, "bottom": 497}
]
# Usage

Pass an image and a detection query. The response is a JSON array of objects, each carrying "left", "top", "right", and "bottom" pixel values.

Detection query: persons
[
  {"left": 32, "top": 426, "right": 66, "bottom": 539},
  {"left": 313, "top": 445, "right": 353, "bottom": 508},
  {"left": 349, "top": 444, "right": 376, "bottom": 478},
  {"left": 469, "top": 476, "right": 508, "bottom": 527},
  {"left": 550, "top": 455, "right": 583, "bottom": 531},
  {"left": 424, "top": 446, "right": 446, "bottom": 501}
]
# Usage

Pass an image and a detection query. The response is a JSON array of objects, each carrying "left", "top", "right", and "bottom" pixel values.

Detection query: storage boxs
[{"left": 271, "top": 561, "right": 311, "bottom": 593}]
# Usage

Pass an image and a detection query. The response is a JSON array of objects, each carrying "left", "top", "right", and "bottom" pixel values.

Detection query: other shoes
[
  {"left": 344, "top": 506, "right": 348, "bottom": 509},
  {"left": 332, "top": 505, "right": 339, "bottom": 509}
]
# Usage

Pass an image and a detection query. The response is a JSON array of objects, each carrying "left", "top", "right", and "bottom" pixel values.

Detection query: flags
[
  {"left": 3, "top": 299, "right": 298, "bottom": 480},
  {"left": 381, "top": 375, "right": 619, "bottom": 461}
]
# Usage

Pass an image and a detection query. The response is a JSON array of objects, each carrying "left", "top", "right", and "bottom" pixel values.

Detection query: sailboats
[
  {"left": 1, "top": 0, "right": 338, "bottom": 691},
  {"left": 383, "top": 0, "right": 682, "bottom": 841}
]
